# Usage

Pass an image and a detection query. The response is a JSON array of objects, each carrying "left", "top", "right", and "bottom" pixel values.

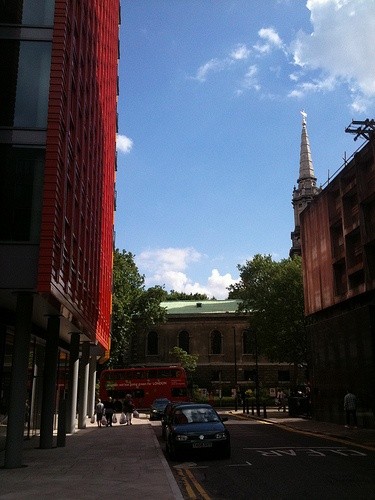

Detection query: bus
[{"left": 99, "top": 365, "right": 189, "bottom": 412}]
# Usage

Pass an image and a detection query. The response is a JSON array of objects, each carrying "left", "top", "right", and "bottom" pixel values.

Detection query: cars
[
  {"left": 150, "top": 398, "right": 172, "bottom": 420},
  {"left": 165, "top": 404, "right": 231, "bottom": 462}
]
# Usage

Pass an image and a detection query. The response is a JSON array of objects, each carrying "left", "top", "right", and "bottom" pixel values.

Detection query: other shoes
[{"left": 344, "top": 425, "right": 350, "bottom": 428}]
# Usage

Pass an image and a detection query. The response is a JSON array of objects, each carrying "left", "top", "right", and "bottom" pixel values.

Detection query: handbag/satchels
[
  {"left": 133, "top": 410, "right": 139, "bottom": 418},
  {"left": 113, "top": 415, "right": 117, "bottom": 423},
  {"left": 90, "top": 416, "right": 95, "bottom": 423},
  {"left": 120, "top": 412, "right": 126, "bottom": 424},
  {"left": 101, "top": 415, "right": 108, "bottom": 425}
]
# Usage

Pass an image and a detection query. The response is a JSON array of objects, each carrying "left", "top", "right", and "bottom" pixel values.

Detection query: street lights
[
  {"left": 231, "top": 325, "right": 238, "bottom": 410},
  {"left": 243, "top": 328, "right": 260, "bottom": 414}
]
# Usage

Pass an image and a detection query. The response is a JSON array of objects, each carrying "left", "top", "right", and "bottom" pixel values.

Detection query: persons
[
  {"left": 277, "top": 388, "right": 286, "bottom": 413},
  {"left": 344, "top": 387, "right": 358, "bottom": 429},
  {"left": 123, "top": 394, "right": 134, "bottom": 425},
  {"left": 104, "top": 397, "right": 114, "bottom": 426},
  {"left": 94, "top": 399, "right": 104, "bottom": 428}
]
